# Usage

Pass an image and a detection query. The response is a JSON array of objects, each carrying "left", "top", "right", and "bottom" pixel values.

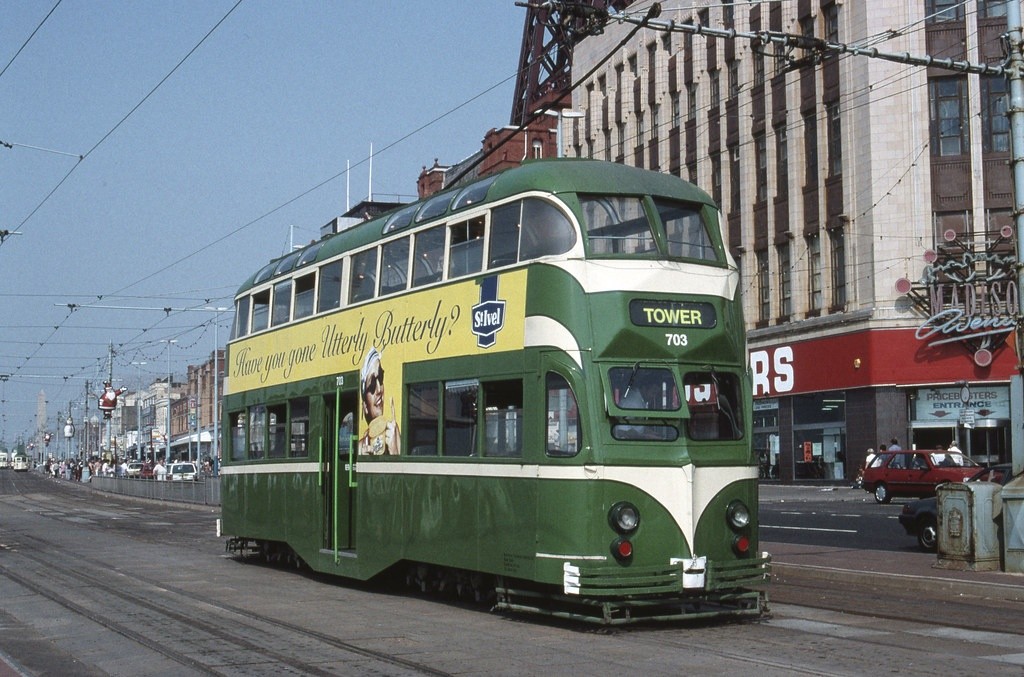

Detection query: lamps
[
  {"left": 735, "top": 246, "right": 746, "bottom": 253},
  {"left": 838, "top": 214, "right": 850, "bottom": 223},
  {"left": 783, "top": 231, "right": 795, "bottom": 239}
]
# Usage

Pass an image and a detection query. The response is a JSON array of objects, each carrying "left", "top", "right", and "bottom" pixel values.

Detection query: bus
[
  {"left": 0, "top": 452, "right": 10, "bottom": 470},
  {"left": 13, "top": 454, "right": 31, "bottom": 471},
  {"left": 219, "top": 158, "right": 777, "bottom": 629}
]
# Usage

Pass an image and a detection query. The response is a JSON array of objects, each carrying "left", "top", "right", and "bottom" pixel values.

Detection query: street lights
[
  {"left": 202, "top": 305, "right": 229, "bottom": 479},
  {"left": 532, "top": 109, "right": 585, "bottom": 157},
  {"left": 131, "top": 362, "right": 149, "bottom": 460},
  {"left": 158, "top": 340, "right": 179, "bottom": 462},
  {"left": 110, "top": 379, "right": 124, "bottom": 475}
]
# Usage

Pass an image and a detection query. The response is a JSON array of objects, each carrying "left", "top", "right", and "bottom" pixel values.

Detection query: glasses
[{"left": 360, "top": 367, "right": 384, "bottom": 395}]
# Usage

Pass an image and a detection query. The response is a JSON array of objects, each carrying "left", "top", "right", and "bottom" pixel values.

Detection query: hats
[{"left": 857, "top": 464, "right": 865, "bottom": 471}]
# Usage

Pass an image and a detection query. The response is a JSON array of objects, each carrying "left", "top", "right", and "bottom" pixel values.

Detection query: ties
[{"left": 642, "top": 400, "right": 648, "bottom": 433}]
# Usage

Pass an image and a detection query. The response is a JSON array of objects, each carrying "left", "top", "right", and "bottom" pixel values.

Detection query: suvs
[{"left": 166, "top": 462, "right": 198, "bottom": 482}]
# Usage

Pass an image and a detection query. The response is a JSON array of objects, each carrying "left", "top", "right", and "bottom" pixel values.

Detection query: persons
[
  {"left": 174, "top": 458, "right": 178, "bottom": 463},
  {"left": 889, "top": 437, "right": 901, "bottom": 462},
  {"left": 98, "top": 383, "right": 127, "bottom": 419},
  {"left": 89, "top": 458, "right": 109, "bottom": 476},
  {"left": 120, "top": 459, "right": 128, "bottom": 477},
  {"left": 865, "top": 449, "right": 878, "bottom": 468},
  {"left": 614, "top": 375, "right": 662, "bottom": 439},
  {"left": 930, "top": 444, "right": 945, "bottom": 465},
  {"left": 358, "top": 347, "right": 401, "bottom": 455},
  {"left": 45, "top": 458, "right": 83, "bottom": 481},
  {"left": 948, "top": 441, "right": 963, "bottom": 466},
  {"left": 204, "top": 456, "right": 213, "bottom": 473},
  {"left": 44, "top": 432, "right": 54, "bottom": 447},
  {"left": 879, "top": 444, "right": 888, "bottom": 459},
  {"left": 152, "top": 459, "right": 166, "bottom": 481}
]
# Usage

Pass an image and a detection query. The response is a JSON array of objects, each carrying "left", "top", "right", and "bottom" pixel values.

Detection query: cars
[
  {"left": 858, "top": 448, "right": 1012, "bottom": 504},
  {"left": 897, "top": 461, "right": 1017, "bottom": 551},
  {"left": 107, "top": 463, "right": 155, "bottom": 477}
]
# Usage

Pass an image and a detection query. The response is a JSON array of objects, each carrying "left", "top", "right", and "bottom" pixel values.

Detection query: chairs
[{"left": 412, "top": 445, "right": 436, "bottom": 458}]
